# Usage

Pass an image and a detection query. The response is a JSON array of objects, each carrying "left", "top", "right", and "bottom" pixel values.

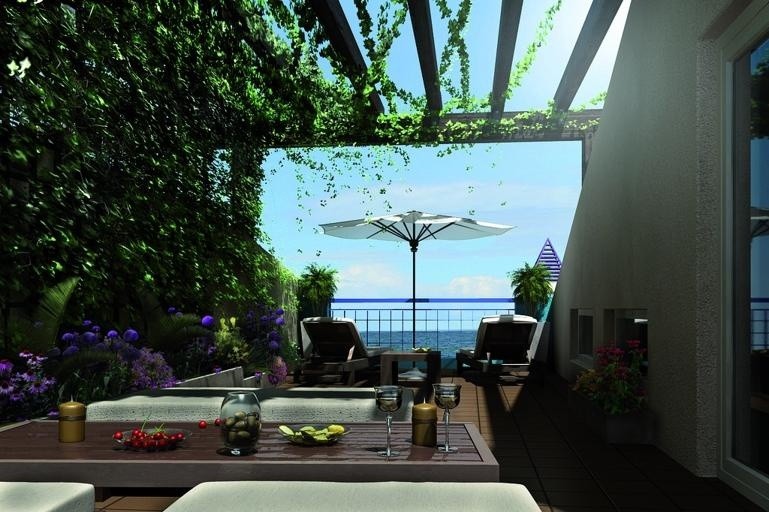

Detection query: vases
[{"left": 592, "top": 408, "right": 657, "bottom": 445}]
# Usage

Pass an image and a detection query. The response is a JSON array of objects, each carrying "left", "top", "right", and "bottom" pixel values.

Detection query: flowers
[{"left": 576, "top": 338, "right": 652, "bottom": 412}]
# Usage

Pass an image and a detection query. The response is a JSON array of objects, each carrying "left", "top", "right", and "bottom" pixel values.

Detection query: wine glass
[
  {"left": 434, "top": 383, "right": 462, "bottom": 454},
  {"left": 374, "top": 385, "right": 404, "bottom": 457}
]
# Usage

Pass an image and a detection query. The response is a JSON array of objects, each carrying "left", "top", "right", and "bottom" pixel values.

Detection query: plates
[
  {"left": 113, "top": 427, "right": 193, "bottom": 453},
  {"left": 278, "top": 422, "right": 352, "bottom": 447}
]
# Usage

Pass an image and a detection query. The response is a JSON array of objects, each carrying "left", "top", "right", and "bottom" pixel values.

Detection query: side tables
[{"left": 380, "top": 350, "right": 441, "bottom": 389}]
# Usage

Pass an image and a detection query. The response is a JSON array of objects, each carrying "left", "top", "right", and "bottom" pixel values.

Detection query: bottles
[{"left": 220, "top": 392, "right": 262, "bottom": 453}]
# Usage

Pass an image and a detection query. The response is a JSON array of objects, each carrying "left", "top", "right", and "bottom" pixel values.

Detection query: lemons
[{"left": 328, "top": 424, "right": 344, "bottom": 435}]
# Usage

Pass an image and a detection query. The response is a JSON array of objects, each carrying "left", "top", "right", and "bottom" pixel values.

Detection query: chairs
[
  {"left": 294, "top": 316, "right": 392, "bottom": 389},
  {"left": 456, "top": 313, "right": 538, "bottom": 383}
]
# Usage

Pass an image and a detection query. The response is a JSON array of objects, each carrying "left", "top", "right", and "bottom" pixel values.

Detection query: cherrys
[
  {"left": 215, "top": 418, "right": 220, "bottom": 425},
  {"left": 113, "top": 429, "right": 184, "bottom": 449},
  {"left": 199, "top": 420, "right": 207, "bottom": 429}
]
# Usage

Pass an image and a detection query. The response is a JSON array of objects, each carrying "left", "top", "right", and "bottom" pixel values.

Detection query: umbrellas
[{"left": 319, "top": 211, "right": 518, "bottom": 368}]
